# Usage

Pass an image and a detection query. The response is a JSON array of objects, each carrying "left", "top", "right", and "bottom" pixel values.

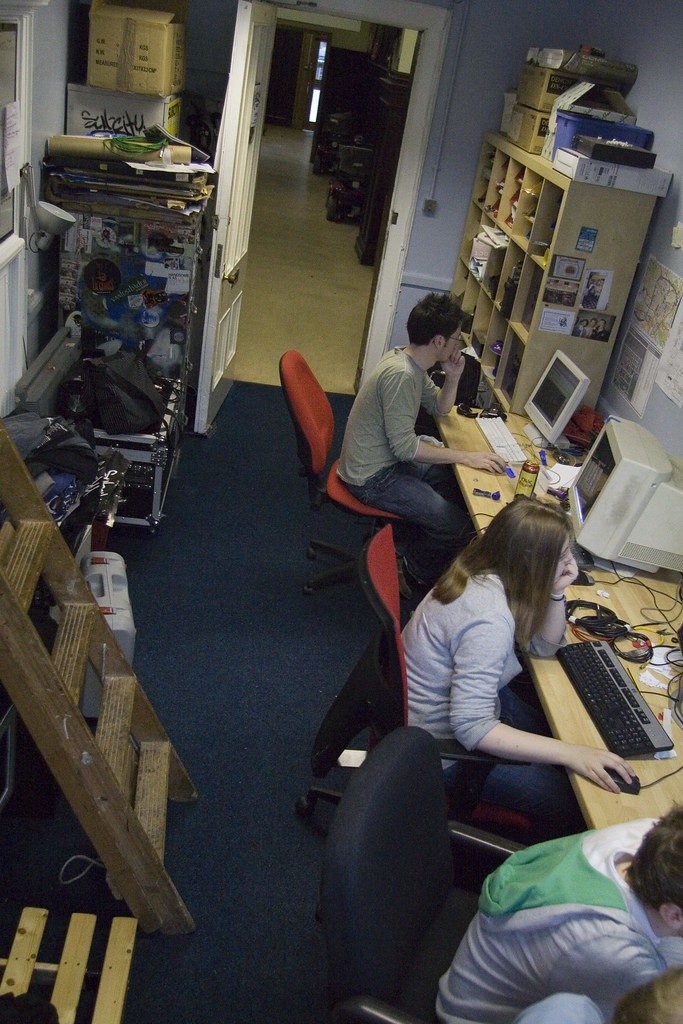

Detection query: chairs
[
  {"left": 295, "top": 523, "right": 535, "bottom": 846},
  {"left": 320, "top": 726, "right": 525, "bottom": 1024},
  {"left": 280, "top": 352, "right": 429, "bottom": 595}
]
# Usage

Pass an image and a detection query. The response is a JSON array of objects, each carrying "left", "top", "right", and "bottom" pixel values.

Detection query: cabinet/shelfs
[
  {"left": 449, "top": 132, "right": 658, "bottom": 415},
  {"left": 58, "top": 211, "right": 204, "bottom": 426}
]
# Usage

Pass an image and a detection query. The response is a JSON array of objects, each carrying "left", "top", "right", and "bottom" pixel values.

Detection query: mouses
[
  {"left": 570, "top": 572, "right": 595, "bottom": 585},
  {"left": 490, "top": 460, "right": 505, "bottom": 472},
  {"left": 603, "top": 767, "right": 640, "bottom": 795}
]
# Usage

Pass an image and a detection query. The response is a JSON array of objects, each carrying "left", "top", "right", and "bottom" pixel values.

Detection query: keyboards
[
  {"left": 554, "top": 640, "right": 674, "bottom": 757},
  {"left": 475, "top": 415, "right": 528, "bottom": 464},
  {"left": 566, "top": 537, "right": 594, "bottom": 568}
]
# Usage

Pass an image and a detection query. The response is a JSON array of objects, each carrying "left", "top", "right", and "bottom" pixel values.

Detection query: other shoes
[{"left": 397, "top": 559, "right": 414, "bottom": 600}]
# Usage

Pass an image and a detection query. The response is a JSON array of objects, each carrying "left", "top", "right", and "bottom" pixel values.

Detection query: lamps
[{"left": 22, "top": 163, "right": 76, "bottom": 253}]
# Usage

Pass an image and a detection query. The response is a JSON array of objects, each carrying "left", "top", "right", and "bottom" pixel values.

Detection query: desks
[{"left": 436, "top": 406, "right": 683, "bottom": 830}]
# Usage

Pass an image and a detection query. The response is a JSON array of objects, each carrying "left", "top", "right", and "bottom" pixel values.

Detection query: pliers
[{"left": 634, "top": 625, "right": 674, "bottom": 646}]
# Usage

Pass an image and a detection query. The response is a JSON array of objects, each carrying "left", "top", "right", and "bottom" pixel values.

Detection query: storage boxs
[
  {"left": 500, "top": 62, "right": 673, "bottom": 197},
  {"left": 92, "top": 378, "right": 181, "bottom": 538},
  {"left": 470, "top": 237, "right": 507, "bottom": 280},
  {"left": 67, "top": 0, "right": 189, "bottom": 138}
]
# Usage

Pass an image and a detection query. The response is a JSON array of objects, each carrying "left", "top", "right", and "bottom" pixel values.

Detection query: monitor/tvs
[
  {"left": 523, "top": 350, "right": 590, "bottom": 450},
  {"left": 568, "top": 415, "right": 682, "bottom": 576}
]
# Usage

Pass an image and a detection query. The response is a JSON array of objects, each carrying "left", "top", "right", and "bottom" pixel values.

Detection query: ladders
[{"left": 2, "top": 422, "right": 203, "bottom": 936}]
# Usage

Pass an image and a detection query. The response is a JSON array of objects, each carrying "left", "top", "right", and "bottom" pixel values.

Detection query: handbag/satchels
[{"left": 83, "top": 350, "right": 166, "bottom": 434}]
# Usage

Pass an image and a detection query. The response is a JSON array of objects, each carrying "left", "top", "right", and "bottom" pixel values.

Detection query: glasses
[{"left": 432, "top": 334, "right": 461, "bottom": 345}]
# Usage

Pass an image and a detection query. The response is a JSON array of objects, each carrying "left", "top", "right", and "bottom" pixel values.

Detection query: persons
[
  {"left": 433, "top": 800, "right": 683, "bottom": 1024},
  {"left": 514, "top": 965, "right": 683, "bottom": 1024},
  {"left": 401, "top": 497, "right": 638, "bottom": 837},
  {"left": 588, "top": 278, "right": 604, "bottom": 298},
  {"left": 572, "top": 317, "right": 610, "bottom": 341},
  {"left": 336, "top": 290, "right": 508, "bottom": 579}
]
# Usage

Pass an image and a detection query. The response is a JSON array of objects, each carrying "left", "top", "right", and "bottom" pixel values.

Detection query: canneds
[{"left": 515, "top": 460, "right": 540, "bottom": 498}]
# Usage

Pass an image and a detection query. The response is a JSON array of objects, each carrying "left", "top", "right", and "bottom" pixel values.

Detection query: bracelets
[
  {"left": 550, "top": 592, "right": 565, "bottom": 597},
  {"left": 548, "top": 594, "right": 565, "bottom": 601}
]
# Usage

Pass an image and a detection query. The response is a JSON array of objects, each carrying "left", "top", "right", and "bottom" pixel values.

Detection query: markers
[{"left": 542, "top": 468, "right": 551, "bottom": 479}]
